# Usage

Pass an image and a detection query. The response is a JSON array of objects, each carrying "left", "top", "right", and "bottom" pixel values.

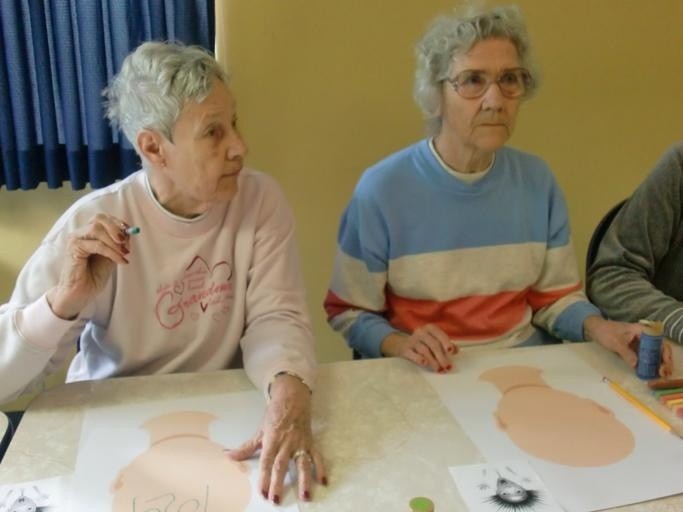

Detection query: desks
[{"left": 1, "top": 335, "right": 683, "bottom": 511}]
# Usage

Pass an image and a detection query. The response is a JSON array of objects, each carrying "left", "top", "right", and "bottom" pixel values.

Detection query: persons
[
  {"left": 586, "top": 146, "right": 683, "bottom": 343},
  {"left": 322, "top": 5, "right": 674, "bottom": 379},
  {"left": 0, "top": 39, "right": 329, "bottom": 505}
]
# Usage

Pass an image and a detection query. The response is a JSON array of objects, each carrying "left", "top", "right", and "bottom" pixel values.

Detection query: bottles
[{"left": 636, "top": 318, "right": 665, "bottom": 380}]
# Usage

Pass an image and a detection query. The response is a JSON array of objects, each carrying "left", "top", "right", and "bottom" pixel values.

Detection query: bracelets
[{"left": 266, "top": 370, "right": 312, "bottom": 405}]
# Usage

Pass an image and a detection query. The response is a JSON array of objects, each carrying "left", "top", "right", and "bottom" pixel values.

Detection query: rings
[{"left": 295, "top": 450, "right": 313, "bottom": 462}]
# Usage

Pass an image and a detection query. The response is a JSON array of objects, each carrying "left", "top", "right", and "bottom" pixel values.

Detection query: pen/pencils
[
  {"left": 602, "top": 376, "right": 682, "bottom": 440},
  {"left": 124, "top": 227, "right": 141, "bottom": 235}
]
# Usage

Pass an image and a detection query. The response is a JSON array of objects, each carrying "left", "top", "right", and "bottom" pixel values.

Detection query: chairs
[{"left": 586, "top": 196, "right": 628, "bottom": 271}]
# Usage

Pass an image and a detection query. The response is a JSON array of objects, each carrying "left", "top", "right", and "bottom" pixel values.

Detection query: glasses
[{"left": 436, "top": 67, "right": 534, "bottom": 99}]
